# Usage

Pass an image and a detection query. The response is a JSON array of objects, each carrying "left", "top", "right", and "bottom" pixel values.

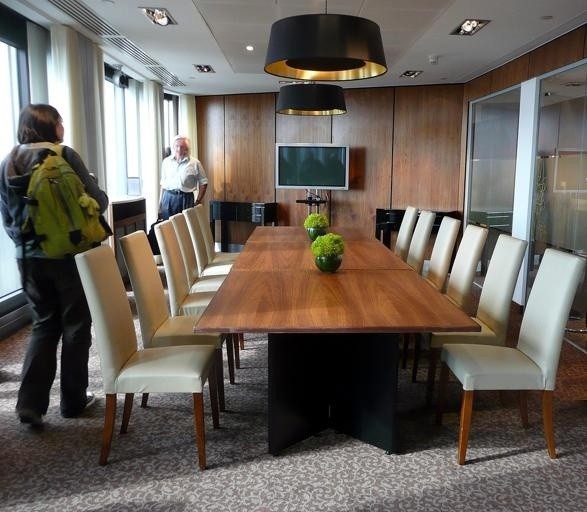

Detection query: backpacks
[{"left": 23, "top": 143, "right": 115, "bottom": 261}]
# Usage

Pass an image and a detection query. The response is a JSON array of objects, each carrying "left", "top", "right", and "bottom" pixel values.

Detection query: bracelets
[{"left": 196, "top": 200, "right": 200, "bottom": 204}]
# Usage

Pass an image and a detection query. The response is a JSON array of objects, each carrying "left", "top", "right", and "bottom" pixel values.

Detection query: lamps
[
  {"left": 275, "top": 82, "right": 347, "bottom": 115},
  {"left": 265, "top": 0, "right": 389, "bottom": 82}
]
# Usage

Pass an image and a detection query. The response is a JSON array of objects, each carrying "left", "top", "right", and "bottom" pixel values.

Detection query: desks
[{"left": 193, "top": 226, "right": 482, "bottom": 455}]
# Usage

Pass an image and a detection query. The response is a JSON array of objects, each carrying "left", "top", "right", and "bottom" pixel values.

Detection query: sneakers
[
  {"left": 60, "top": 390, "right": 97, "bottom": 420},
  {"left": 17, "top": 407, "right": 45, "bottom": 429}
]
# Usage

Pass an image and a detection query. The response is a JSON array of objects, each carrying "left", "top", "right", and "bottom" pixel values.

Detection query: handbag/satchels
[{"left": 148, "top": 217, "right": 168, "bottom": 255}]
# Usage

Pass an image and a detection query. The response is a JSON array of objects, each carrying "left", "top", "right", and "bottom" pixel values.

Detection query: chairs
[
  {"left": 153, "top": 203, "right": 245, "bottom": 371},
  {"left": 74, "top": 243, "right": 220, "bottom": 470},
  {"left": 437, "top": 247, "right": 587, "bottom": 465},
  {"left": 394, "top": 205, "right": 489, "bottom": 311},
  {"left": 118, "top": 229, "right": 234, "bottom": 412},
  {"left": 411, "top": 233, "right": 528, "bottom": 414}
]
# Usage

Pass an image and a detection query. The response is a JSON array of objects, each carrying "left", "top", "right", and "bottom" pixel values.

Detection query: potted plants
[
  {"left": 310, "top": 232, "right": 346, "bottom": 273},
  {"left": 304, "top": 213, "right": 329, "bottom": 240}
]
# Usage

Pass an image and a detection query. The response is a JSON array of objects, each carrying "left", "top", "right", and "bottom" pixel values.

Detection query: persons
[
  {"left": 157, "top": 135, "right": 208, "bottom": 222},
  {"left": 0, "top": 104, "right": 106, "bottom": 426}
]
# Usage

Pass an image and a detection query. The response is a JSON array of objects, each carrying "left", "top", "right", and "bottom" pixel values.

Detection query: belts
[{"left": 166, "top": 188, "right": 193, "bottom": 194}]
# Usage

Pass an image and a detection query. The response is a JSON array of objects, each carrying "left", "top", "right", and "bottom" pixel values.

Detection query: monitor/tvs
[
  {"left": 553, "top": 148, "right": 587, "bottom": 192},
  {"left": 275, "top": 142, "right": 349, "bottom": 191}
]
